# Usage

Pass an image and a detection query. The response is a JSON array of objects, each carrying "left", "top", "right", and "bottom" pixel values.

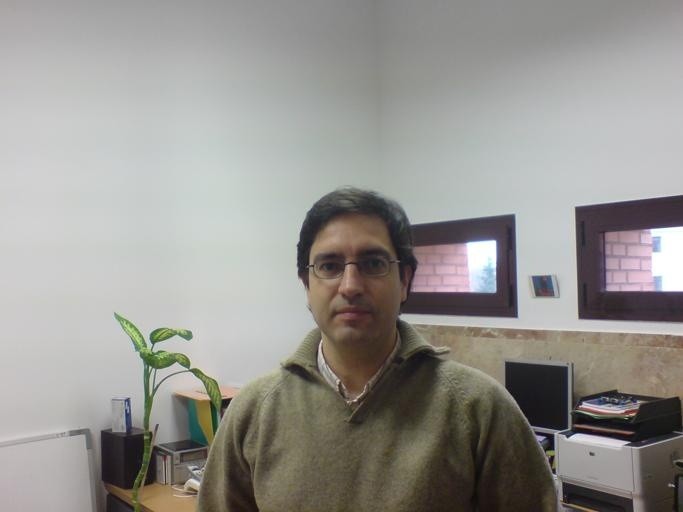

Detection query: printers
[{"left": 554, "top": 428, "right": 683, "bottom": 512}]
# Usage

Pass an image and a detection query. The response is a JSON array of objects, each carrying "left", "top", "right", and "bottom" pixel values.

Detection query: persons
[{"left": 198, "top": 187, "right": 558, "bottom": 512}]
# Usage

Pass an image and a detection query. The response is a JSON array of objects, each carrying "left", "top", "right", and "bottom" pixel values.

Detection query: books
[{"left": 570, "top": 396, "right": 650, "bottom": 420}]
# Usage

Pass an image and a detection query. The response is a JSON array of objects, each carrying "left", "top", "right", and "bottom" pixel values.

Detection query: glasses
[{"left": 305, "top": 255, "right": 401, "bottom": 280}]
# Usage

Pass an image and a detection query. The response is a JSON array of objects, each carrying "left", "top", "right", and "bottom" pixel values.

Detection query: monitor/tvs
[{"left": 501, "top": 356, "right": 574, "bottom": 435}]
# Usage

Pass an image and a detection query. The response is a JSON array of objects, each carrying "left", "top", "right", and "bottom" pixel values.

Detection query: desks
[{"left": 100, "top": 472, "right": 203, "bottom": 512}]
